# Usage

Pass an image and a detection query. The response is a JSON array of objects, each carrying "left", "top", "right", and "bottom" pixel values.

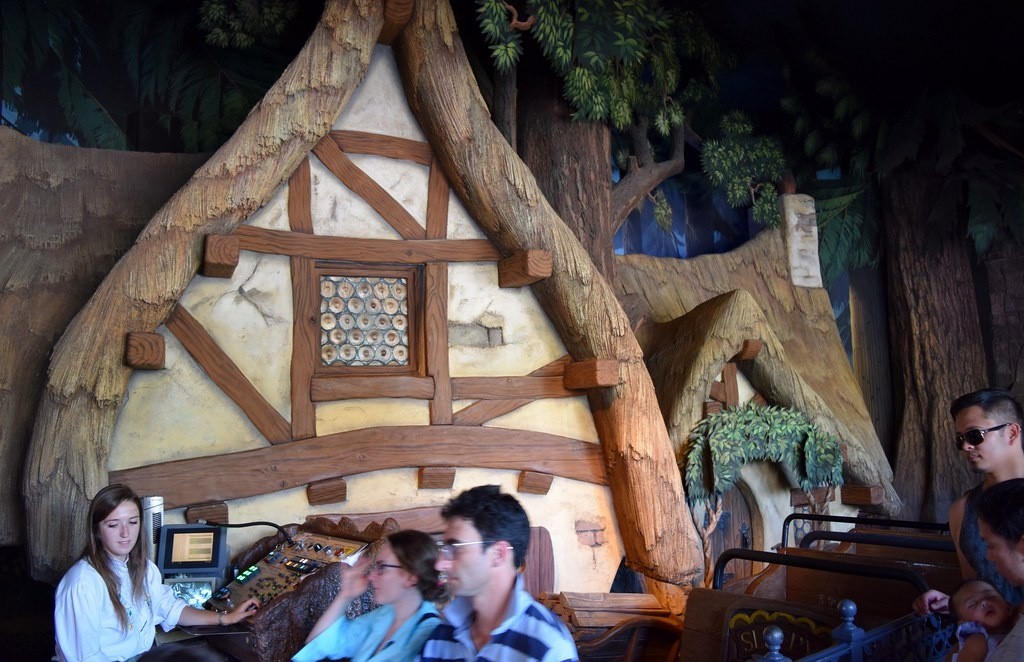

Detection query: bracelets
[{"left": 219, "top": 611, "right": 228, "bottom": 626}]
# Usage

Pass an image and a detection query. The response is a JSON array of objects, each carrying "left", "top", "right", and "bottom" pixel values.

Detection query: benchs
[
  {"left": 746, "top": 547, "right": 965, "bottom": 626},
  {"left": 829, "top": 528, "right": 955, "bottom": 562},
  {"left": 579, "top": 587, "right": 877, "bottom": 662}
]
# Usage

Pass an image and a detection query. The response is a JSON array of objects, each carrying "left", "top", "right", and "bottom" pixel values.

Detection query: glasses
[
  {"left": 955, "top": 422, "right": 1022, "bottom": 451},
  {"left": 436, "top": 539, "right": 499, "bottom": 561},
  {"left": 374, "top": 560, "right": 405, "bottom": 575}
]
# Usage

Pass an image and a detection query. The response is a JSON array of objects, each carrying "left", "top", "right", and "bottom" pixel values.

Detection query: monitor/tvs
[{"left": 158, "top": 524, "right": 227, "bottom": 577}]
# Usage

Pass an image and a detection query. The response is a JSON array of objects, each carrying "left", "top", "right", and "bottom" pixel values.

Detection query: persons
[
  {"left": 948, "top": 579, "right": 1017, "bottom": 662},
  {"left": 292, "top": 529, "right": 442, "bottom": 662},
  {"left": 912, "top": 389, "right": 1024, "bottom": 615},
  {"left": 944, "top": 478, "right": 1024, "bottom": 662},
  {"left": 416, "top": 484, "right": 578, "bottom": 662},
  {"left": 52, "top": 484, "right": 260, "bottom": 662}
]
